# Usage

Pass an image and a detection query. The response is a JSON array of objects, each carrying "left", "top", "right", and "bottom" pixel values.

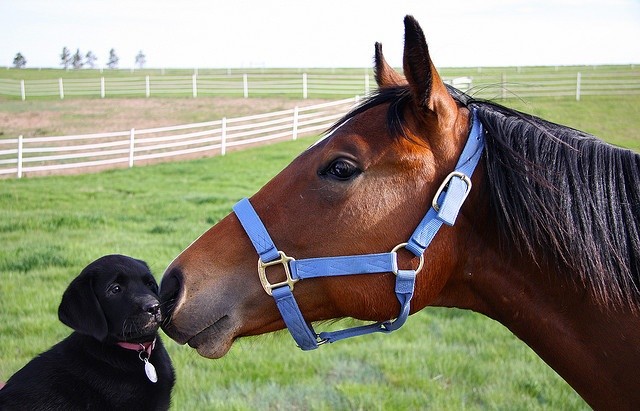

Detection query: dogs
[{"left": 0, "top": 252, "right": 178, "bottom": 409}]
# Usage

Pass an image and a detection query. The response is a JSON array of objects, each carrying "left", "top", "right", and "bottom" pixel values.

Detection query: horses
[{"left": 158, "top": 12, "right": 640, "bottom": 410}]
